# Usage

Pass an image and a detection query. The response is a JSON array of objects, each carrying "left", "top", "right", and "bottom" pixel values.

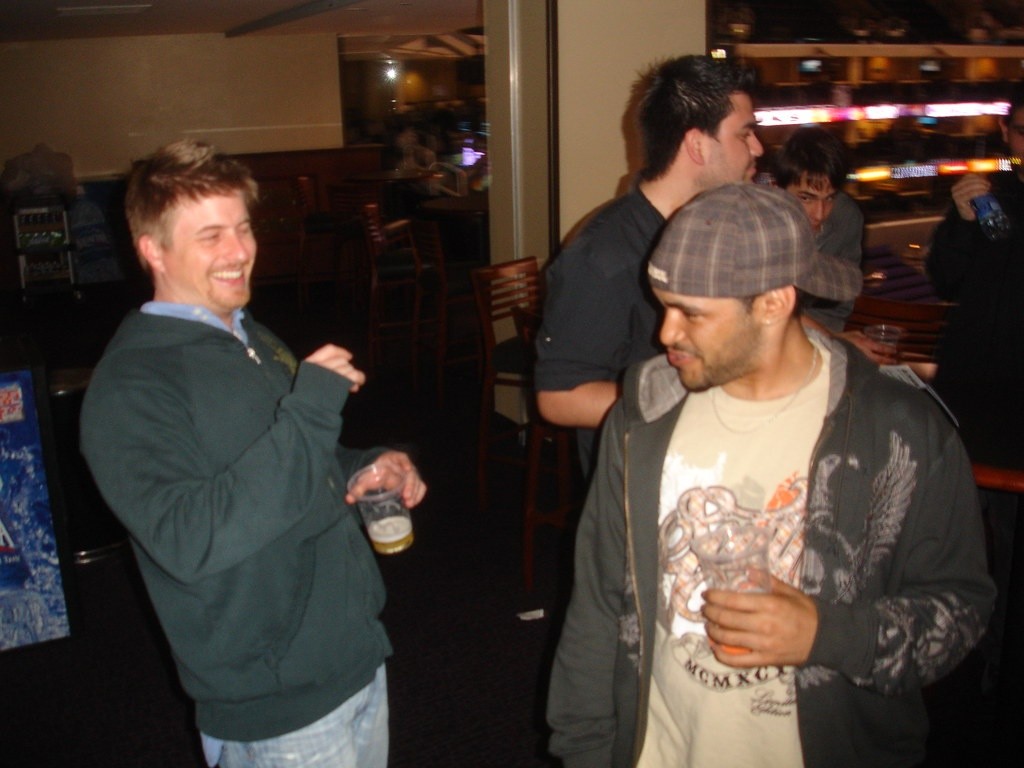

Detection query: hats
[{"left": 646, "top": 181, "right": 864, "bottom": 301}]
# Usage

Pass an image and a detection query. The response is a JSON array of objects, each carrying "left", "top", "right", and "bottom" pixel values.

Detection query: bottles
[{"left": 969, "top": 194, "right": 1009, "bottom": 242}]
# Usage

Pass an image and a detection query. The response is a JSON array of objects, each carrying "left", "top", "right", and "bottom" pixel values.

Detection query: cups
[
  {"left": 346, "top": 462, "right": 414, "bottom": 555},
  {"left": 690, "top": 525, "right": 772, "bottom": 656},
  {"left": 864, "top": 325, "right": 901, "bottom": 357}
]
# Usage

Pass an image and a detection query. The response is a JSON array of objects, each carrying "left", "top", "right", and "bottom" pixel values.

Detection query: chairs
[{"left": 290, "top": 169, "right": 1024, "bottom": 596}]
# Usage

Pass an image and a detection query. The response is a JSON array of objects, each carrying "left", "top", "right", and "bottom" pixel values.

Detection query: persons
[
  {"left": 926, "top": 77, "right": 1024, "bottom": 669},
  {"left": 375, "top": 122, "right": 437, "bottom": 231},
  {"left": 81, "top": 145, "right": 429, "bottom": 768},
  {"left": 778, "top": 129, "right": 898, "bottom": 365},
  {"left": 535, "top": 57, "right": 764, "bottom": 485},
  {"left": 545, "top": 184, "right": 996, "bottom": 767}
]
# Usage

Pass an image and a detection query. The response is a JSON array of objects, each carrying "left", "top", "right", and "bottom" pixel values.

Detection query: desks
[
  {"left": 330, "top": 166, "right": 422, "bottom": 222},
  {"left": 416, "top": 194, "right": 490, "bottom": 263}
]
